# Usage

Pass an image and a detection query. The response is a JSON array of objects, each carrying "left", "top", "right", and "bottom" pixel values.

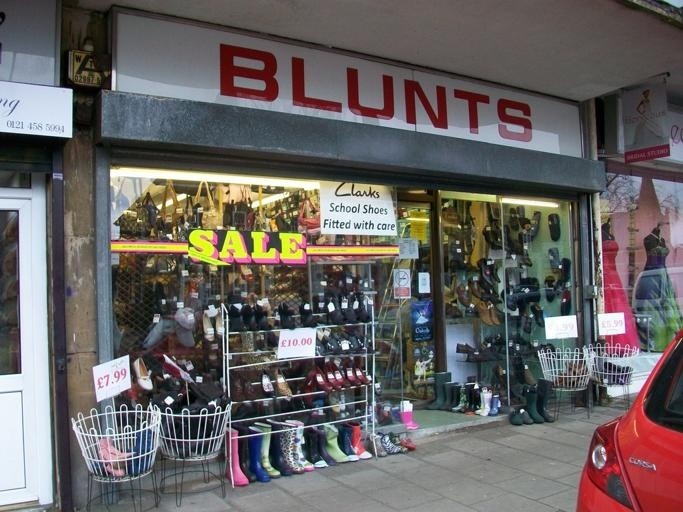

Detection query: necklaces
[
  {"left": 650, "top": 232, "right": 663, "bottom": 242},
  {"left": 606, "top": 233, "right": 611, "bottom": 240}
]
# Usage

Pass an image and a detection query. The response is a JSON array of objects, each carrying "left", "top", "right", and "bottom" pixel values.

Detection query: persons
[
  {"left": 601, "top": 218, "right": 640, "bottom": 352},
  {"left": 635, "top": 222, "right": 682, "bottom": 352},
  {"left": 633, "top": 89, "right": 664, "bottom": 148}
]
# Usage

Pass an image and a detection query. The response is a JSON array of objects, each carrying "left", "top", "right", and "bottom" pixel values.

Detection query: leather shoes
[
  {"left": 447, "top": 206, "right": 545, "bottom": 333},
  {"left": 315, "top": 332, "right": 364, "bottom": 355},
  {"left": 548, "top": 214, "right": 560, "bottom": 241},
  {"left": 134, "top": 358, "right": 154, "bottom": 391},
  {"left": 261, "top": 367, "right": 292, "bottom": 396},
  {"left": 456, "top": 334, "right": 558, "bottom": 385},
  {"left": 545, "top": 248, "right": 571, "bottom": 315},
  {"left": 314, "top": 361, "right": 370, "bottom": 412},
  {"left": 155, "top": 384, "right": 229, "bottom": 459}
]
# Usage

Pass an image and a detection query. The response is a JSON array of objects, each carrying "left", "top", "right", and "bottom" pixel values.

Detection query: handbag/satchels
[{"left": 114, "top": 181, "right": 319, "bottom": 238}]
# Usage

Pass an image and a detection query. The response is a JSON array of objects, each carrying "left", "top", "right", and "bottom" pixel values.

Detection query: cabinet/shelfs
[
  {"left": 220, "top": 297, "right": 377, "bottom": 488},
  {"left": 444, "top": 317, "right": 532, "bottom": 365}
]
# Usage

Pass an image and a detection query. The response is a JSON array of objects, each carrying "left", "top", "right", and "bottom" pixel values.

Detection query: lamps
[{"left": 501, "top": 197, "right": 560, "bottom": 208}]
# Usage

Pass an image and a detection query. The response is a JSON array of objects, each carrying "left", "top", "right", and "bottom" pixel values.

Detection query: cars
[{"left": 575, "top": 327, "right": 683, "bottom": 511}]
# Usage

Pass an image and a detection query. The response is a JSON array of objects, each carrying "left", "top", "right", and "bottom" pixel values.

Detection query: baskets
[
  {"left": 69, "top": 404, "right": 161, "bottom": 481},
  {"left": 537, "top": 347, "right": 595, "bottom": 390},
  {"left": 583, "top": 343, "right": 638, "bottom": 385},
  {"left": 153, "top": 404, "right": 229, "bottom": 460}
]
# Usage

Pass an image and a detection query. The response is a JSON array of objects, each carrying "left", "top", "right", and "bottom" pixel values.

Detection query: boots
[
  {"left": 428, "top": 372, "right": 499, "bottom": 416},
  {"left": 224, "top": 420, "right": 372, "bottom": 486},
  {"left": 368, "top": 433, "right": 416, "bottom": 457},
  {"left": 229, "top": 372, "right": 256, "bottom": 419},
  {"left": 203, "top": 304, "right": 273, "bottom": 341},
  {"left": 176, "top": 309, "right": 197, "bottom": 348},
  {"left": 92, "top": 433, "right": 153, "bottom": 475},
  {"left": 279, "top": 305, "right": 294, "bottom": 328},
  {"left": 512, "top": 380, "right": 554, "bottom": 425},
  {"left": 300, "top": 303, "right": 317, "bottom": 326},
  {"left": 324, "top": 294, "right": 369, "bottom": 325},
  {"left": 377, "top": 400, "right": 417, "bottom": 430}
]
